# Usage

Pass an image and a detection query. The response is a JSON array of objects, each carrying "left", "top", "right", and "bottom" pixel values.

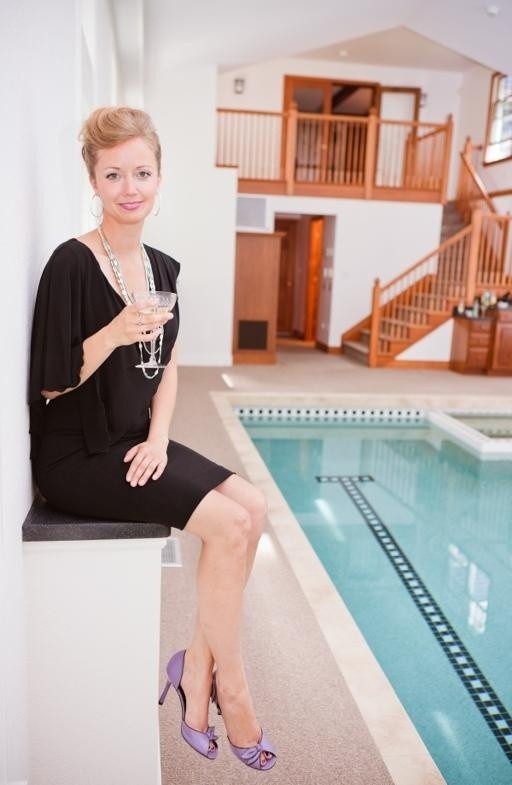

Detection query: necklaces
[{"left": 97, "top": 224, "right": 169, "bottom": 379}]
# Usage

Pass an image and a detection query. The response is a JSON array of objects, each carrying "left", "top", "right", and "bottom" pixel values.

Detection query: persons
[{"left": 29, "top": 106, "right": 282, "bottom": 772}]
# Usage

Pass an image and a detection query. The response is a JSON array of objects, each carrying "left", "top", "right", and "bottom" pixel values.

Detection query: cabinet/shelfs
[
  {"left": 234, "top": 233, "right": 287, "bottom": 365},
  {"left": 444, "top": 299, "right": 511, "bottom": 378}
]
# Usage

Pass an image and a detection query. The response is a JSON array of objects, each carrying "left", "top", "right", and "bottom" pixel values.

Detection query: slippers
[{"left": 207, "top": 668, "right": 279, "bottom": 772}]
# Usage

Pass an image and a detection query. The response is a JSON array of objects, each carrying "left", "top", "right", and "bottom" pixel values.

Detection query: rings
[{"left": 138, "top": 313, "right": 143, "bottom": 325}]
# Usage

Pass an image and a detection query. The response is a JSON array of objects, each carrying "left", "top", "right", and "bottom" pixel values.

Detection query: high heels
[{"left": 158, "top": 647, "right": 219, "bottom": 762}]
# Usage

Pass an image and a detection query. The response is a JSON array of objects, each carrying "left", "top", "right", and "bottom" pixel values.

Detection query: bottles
[{"left": 455, "top": 290, "right": 509, "bottom": 318}]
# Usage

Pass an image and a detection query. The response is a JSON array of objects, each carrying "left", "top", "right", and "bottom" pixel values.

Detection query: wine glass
[{"left": 131, "top": 290, "right": 180, "bottom": 369}]
